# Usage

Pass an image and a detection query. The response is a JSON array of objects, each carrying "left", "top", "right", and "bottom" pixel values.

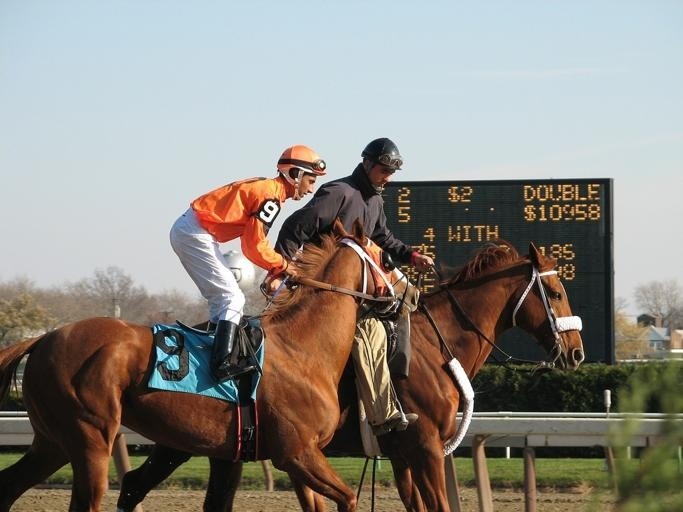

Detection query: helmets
[
  {"left": 362, "top": 138, "right": 402, "bottom": 169},
  {"left": 277, "top": 145, "right": 327, "bottom": 175}
]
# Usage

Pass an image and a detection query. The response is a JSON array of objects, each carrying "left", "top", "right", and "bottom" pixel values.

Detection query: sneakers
[{"left": 372, "top": 413, "right": 418, "bottom": 436}]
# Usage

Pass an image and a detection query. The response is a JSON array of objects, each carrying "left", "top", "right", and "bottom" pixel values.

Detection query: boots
[{"left": 210, "top": 320, "right": 255, "bottom": 383}]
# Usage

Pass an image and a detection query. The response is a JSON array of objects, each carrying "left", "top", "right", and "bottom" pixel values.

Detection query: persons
[
  {"left": 170, "top": 145, "right": 326, "bottom": 382},
  {"left": 263, "top": 138, "right": 434, "bottom": 436}
]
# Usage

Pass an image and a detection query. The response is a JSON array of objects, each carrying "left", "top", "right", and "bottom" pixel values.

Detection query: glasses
[
  {"left": 379, "top": 155, "right": 402, "bottom": 168},
  {"left": 278, "top": 159, "right": 325, "bottom": 172}
]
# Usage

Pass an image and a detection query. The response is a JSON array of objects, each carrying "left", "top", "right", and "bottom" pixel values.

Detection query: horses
[
  {"left": 117, "top": 239, "right": 587, "bottom": 512},
  {"left": 0, "top": 215, "right": 421, "bottom": 512}
]
